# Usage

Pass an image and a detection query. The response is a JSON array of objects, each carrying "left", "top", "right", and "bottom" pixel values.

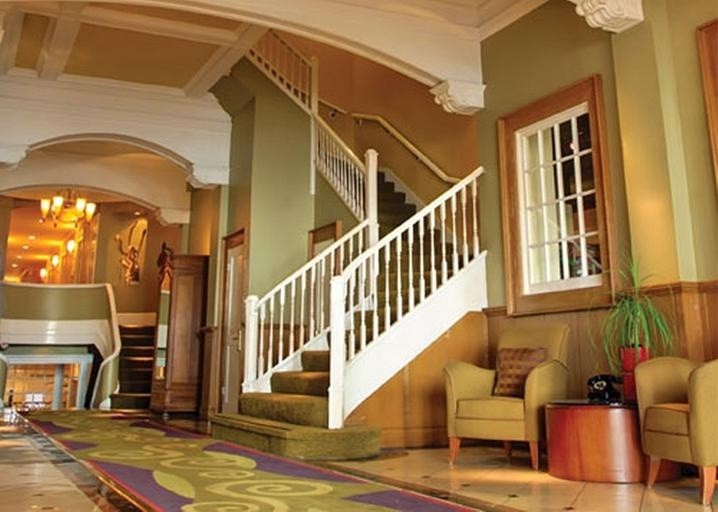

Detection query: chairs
[
  {"left": 442, "top": 323, "right": 572, "bottom": 470},
  {"left": 631, "top": 354, "right": 718, "bottom": 502}
]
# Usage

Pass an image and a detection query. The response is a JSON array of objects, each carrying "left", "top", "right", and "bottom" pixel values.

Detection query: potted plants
[{"left": 594, "top": 243, "right": 675, "bottom": 402}]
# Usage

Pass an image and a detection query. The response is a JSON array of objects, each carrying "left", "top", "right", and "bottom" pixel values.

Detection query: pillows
[{"left": 493, "top": 348, "right": 545, "bottom": 397}]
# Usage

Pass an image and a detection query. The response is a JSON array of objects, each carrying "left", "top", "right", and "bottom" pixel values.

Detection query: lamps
[{"left": 35, "top": 186, "right": 99, "bottom": 227}]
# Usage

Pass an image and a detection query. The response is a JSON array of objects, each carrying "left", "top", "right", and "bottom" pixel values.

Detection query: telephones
[{"left": 587, "top": 374, "right": 622, "bottom": 405}]
